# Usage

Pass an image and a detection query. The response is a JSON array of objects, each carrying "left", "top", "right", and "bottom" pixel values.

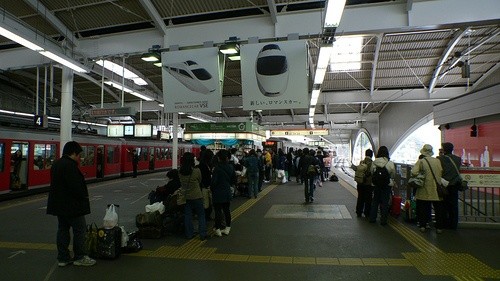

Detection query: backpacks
[
  {"left": 372, "top": 166, "right": 390, "bottom": 187},
  {"left": 354, "top": 164, "right": 367, "bottom": 183}
]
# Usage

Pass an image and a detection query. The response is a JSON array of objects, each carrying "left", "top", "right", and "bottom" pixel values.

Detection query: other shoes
[
  {"left": 58, "top": 259, "right": 72, "bottom": 266},
  {"left": 212, "top": 227, "right": 222, "bottom": 236},
  {"left": 223, "top": 227, "right": 232, "bottom": 235},
  {"left": 355, "top": 212, "right": 362, "bottom": 217},
  {"left": 380, "top": 223, "right": 388, "bottom": 226},
  {"left": 369, "top": 220, "right": 376, "bottom": 224},
  {"left": 418, "top": 227, "right": 426, "bottom": 232},
  {"left": 73, "top": 256, "right": 96, "bottom": 266},
  {"left": 437, "top": 229, "right": 443, "bottom": 234}
]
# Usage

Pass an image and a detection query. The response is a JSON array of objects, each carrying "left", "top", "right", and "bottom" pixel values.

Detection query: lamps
[
  {"left": 0, "top": 24, "right": 213, "bottom": 124},
  {"left": 306, "top": 0, "right": 366, "bottom": 129},
  {"left": 218, "top": 39, "right": 242, "bottom": 63}
]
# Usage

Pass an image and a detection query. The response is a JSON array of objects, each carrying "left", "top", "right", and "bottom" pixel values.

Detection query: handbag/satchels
[
  {"left": 87, "top": 202, "right": 173, "bottom": 257},
  {"left": 307, "top": 165, "right": 317, "bottom": 177},
  {"left": 457, "top": 178, "right": 468, "bottom": 191},
  {"left": 274, "top": 169, "right": 289, "bottom": 184},
  {"left": 330, "top": 175, "right": 338, "bottom": 181},
  {"left": 436, "top": 183, "right": 449, "bottom": 197}
]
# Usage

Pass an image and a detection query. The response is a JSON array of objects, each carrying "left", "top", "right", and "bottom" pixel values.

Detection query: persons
[
  {"left": 155, "top": 145, "right": 241, "bottom": 240},
  {"left": 11, "top": 149, "right": 22, "bottom": 183},
  {"left": 411, "top": 142, "right": 462, "bottom": 233},
  {"left": 140, "top": 148, "right": 172, "bottom": 161},
  {"left": 35, "top": 155, "right": 53, "bottom": 168},
  {"left": 241, "top": 145, "right": 332, "bottom": 203},
  {"left": 132, "top": 150, "right": 139, "bottom": 178},
  {"left": 354, "top": 146, "right": 396, "bottom": 226},
  {"left": 97, "top": 149, "right": 103, "bottom": 178},
  {"left": 46, "top": 141, "right": 96, "bottom": 266}
]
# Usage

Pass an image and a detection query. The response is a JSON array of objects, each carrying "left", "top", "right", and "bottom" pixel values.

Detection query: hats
[
  {"left": 441, "top": 142, "right": 454, "bottom": 151},
  {"left": 420, "top": 144, "right": 434, "bottom": 157}
]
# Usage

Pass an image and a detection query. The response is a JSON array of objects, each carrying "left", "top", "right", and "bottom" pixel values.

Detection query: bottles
[{"left": 396, "top": 165, "right": 401, "bottom": 174}]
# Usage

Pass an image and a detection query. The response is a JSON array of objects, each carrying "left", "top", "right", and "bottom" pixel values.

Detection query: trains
[{"left": 0, "top": 114, "right": 207, "bottom": 203}]
